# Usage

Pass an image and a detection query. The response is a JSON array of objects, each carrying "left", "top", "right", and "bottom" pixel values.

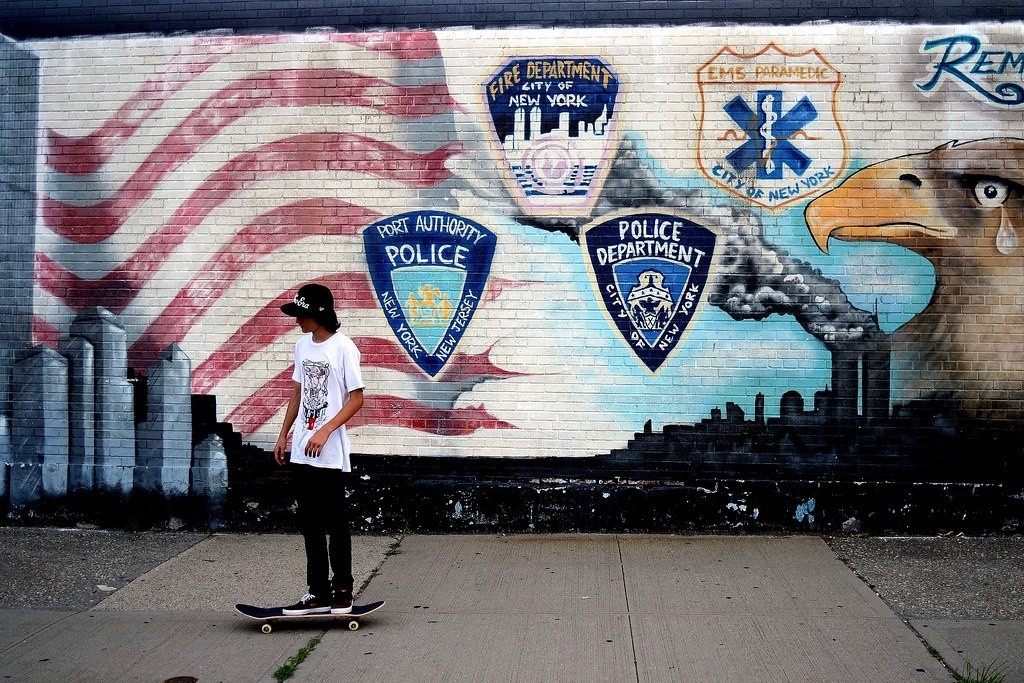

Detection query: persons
[{"left": 273, "top": 283, "right": 368, "bottom": 616}]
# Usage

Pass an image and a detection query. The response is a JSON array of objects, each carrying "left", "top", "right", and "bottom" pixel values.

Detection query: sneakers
[
  {"left": 282, "top": 594, "right": 331, "bottom": 615},
  {"left": 331, "top": 589, "right": 354, "bottom": 613}
]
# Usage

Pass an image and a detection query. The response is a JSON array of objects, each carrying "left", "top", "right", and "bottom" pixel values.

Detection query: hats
[{"left": 280, "top": 284, "right": 334, "bottom": 316}]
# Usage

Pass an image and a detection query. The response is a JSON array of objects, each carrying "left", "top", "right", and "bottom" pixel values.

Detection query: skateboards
[{"left": 234, "top": 601, "right": 386, "bottom": 633}]
